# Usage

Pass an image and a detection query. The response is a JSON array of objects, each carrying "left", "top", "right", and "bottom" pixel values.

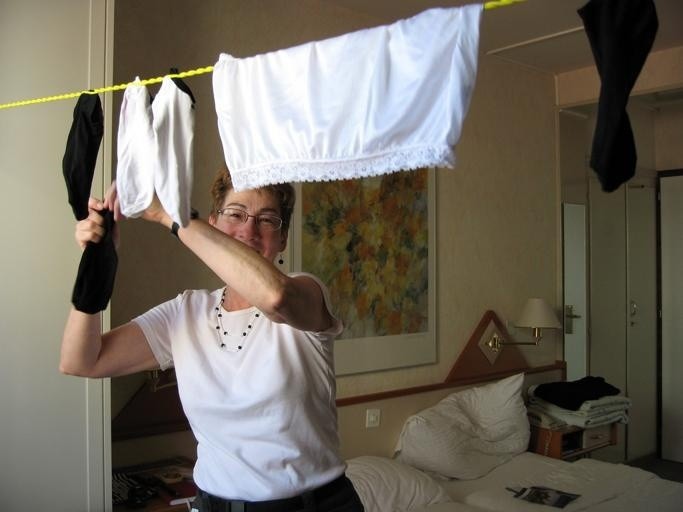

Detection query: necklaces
[{"left": 215, "top": 289, "right": 260, "bottom": 350}]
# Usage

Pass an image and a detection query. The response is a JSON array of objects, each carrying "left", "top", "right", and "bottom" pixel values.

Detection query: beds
[{"left": 325, "top": 373, "right": 682, "bottom": 512}]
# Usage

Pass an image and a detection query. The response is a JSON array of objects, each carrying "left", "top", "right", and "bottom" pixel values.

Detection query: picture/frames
[{"left": 288, "top": 162, "right": 438, "bottom": 380}]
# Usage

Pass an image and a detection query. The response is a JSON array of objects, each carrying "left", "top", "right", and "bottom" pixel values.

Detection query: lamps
[{"left": 475, "top": 295, "right": 563, "bottom": 368}]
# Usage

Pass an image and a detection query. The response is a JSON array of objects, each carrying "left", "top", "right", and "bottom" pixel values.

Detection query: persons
[{"left": 59, "top": 161, "right": 366, "bottom": 512}]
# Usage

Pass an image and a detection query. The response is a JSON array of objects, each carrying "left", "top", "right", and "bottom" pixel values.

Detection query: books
[{"left": 155, "top": 483, "right": 200, "bottom": 506}]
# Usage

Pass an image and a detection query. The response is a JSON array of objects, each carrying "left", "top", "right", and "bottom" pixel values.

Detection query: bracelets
[{"left": 171, "top": 206, "right": 199, "bottom": 236}]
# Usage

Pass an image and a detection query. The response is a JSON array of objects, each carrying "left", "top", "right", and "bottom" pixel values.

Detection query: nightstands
[
  {"left": 518, "top": 407, "right": 624, "bottom": 463},
  {"left": 111, "top": 455, "right": 200, "bottom": 511}
]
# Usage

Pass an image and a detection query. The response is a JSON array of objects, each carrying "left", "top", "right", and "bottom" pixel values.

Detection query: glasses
[{"left": 215, "top": 208, "right": 287, "bottom": 232}]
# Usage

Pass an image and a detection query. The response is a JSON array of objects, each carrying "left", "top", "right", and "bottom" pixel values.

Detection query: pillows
[
  {"left": 386, "top": 370, "right": 534, "bottom": 480},
  {"left": 338, "top": 452, "right": 459, "bottom": 511}
]
